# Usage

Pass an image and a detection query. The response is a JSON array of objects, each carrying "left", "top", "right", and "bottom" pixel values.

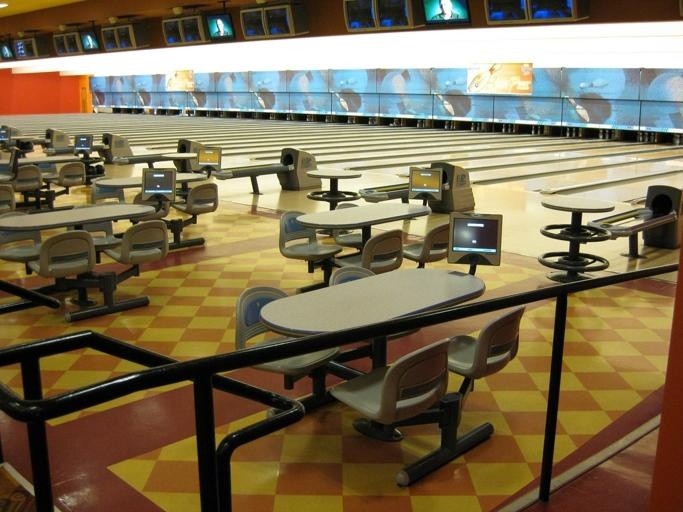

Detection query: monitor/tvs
[
  {"left": 0, "top": 41, "right": 16, "bottom": 61},
  {"left": 421, "top": 0, "right": 472, "bottom": 25},
  {"left": 79, "top": 31, "right": 101, "bottom": 52},
  {"left": 0, "top": 125, "right": 11, "bottom": 141},
  {"left": 9, "top": 146, "right": 20, "bottom": 173},
  {"left": 447, "top": 211, "right": 502, "bottom": 266},
  {"left": 409, "top": 167, "right": 442, "bottom": 200},
  {"left": 142, "top": 168, "right": 176, "bottom": 201},
  {"left": 197, "top": 146, "right": 222, "bottom": 171},
  {"left": 205, "top": 12, "right": 236, "bottom": 41},
  {"left": 73, "top": 134, "right": 93, "bottom": 154}
]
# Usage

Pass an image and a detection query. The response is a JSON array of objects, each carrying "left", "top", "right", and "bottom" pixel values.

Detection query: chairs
[
  {"left": 235, "top": 287, "right": 336, "bottom": 371},
  {"left": 331, "top": 339, "right": 451, "bottom": 426},
  {"left": 447, "top": 306, "right": 526, "bottom": 380},
  {"left": 330, "top": 267, "right": 419, "bottom": 341},
  {"left": 1, "top": 123, "right": 219, "bottom": 321},
  {"left": 404, "top": 223, "right": 449, "bottom": 263},
  {"left": 330, "top": 231, "right": 406, "bottom": 274},
  {"left": 334, "top": 203, "right": 373, "bottom": 248},
  {"left": 279, "top": 211, "right": 336, "bottom": 258}
]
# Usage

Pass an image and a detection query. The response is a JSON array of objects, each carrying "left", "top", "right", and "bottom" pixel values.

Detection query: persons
[
  {"left": 213, "top": 18, "right": 230, "bottom": 37},
  {"left": 4, "top": 46, "right": 13, "bottom": 58},
  {"left": 431, "top": 0, "right": 462, "bottom": 20},
  {"left": 85, "top": 35, "right": 96, "bottom": 49}
]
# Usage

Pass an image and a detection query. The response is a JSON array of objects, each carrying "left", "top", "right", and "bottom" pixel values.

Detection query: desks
[
  {"left": 257, "top": 269, "right": 484, "bottom": 484},
  {"left": 294, "top": 200, "right": 431, "bottom": 295}
]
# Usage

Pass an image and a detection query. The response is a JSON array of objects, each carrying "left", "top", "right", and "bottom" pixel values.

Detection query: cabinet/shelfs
[
  {"left": 539, "top": 195, "right": 615, "bottom": 283},
  {"left": 305, "top": 170, "right": 361, "bottom": 235}
]
